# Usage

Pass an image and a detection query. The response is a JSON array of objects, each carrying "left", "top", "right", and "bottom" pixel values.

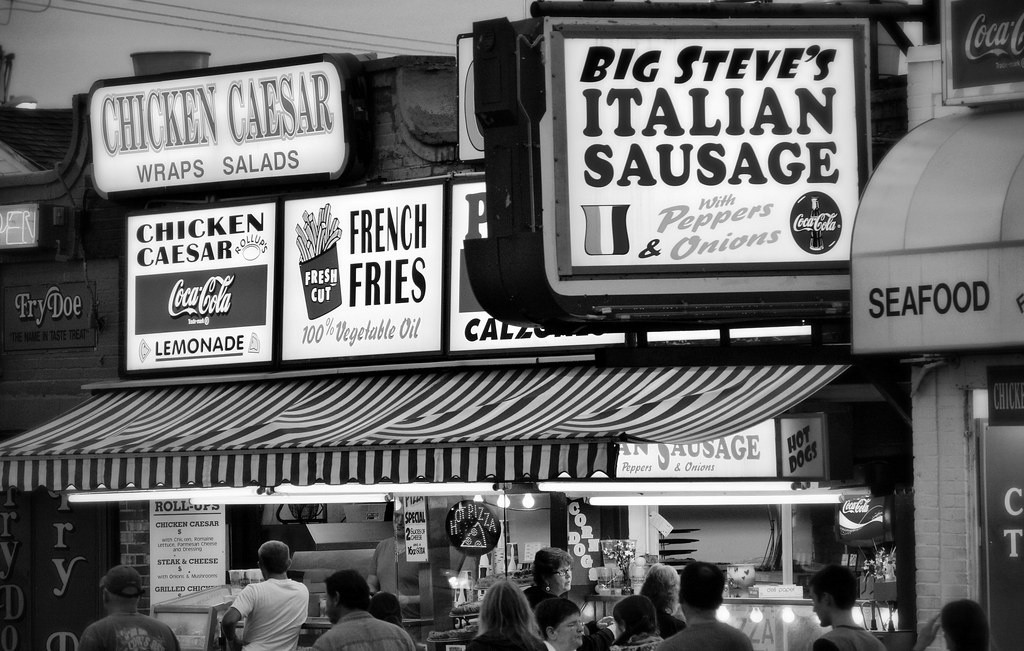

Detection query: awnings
[{"left": 0, "top": 364, "right": 854, "bottom": 491}]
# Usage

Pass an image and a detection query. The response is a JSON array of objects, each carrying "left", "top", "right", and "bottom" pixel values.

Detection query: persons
[
  {"left": 75, "top": 565, "right": 181, "bottom": 651},
  {"left": 464, "top": 580, "right": 550, "bottom": 651},
  {"left": 810, "top": 565, "right": 890, "bottom": 651},
  {"left": 370, "top": 590, "right": 402, "bottom": 628},
  {"left": 522, "top": 547, "right": 688, "bottom": 651},
  {"left": 222, "top": 540, "right": 309, "bottom": 651},
  {"left": 657, "top": 561, "right": 756, "bottom": 651},
  {"left": 309, "top": 569, "right": 417, "bottom": 651},
  {"left": 910, "top": 600, "right": 990, "bottom": 651},
  {"left": 364, "top": 497, "right": 420, "bottom": 645}
]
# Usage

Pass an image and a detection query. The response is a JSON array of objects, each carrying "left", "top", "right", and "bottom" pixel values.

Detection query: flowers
[{"left": 601, "top": 538, "right": 639, "bottom": 572}]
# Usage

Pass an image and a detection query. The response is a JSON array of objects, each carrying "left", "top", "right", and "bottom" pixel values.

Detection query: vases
[{"left": 621, "top": 575, "right": 632, "bottom": 587}]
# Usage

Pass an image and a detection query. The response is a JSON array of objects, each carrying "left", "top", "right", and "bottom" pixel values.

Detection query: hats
[{"left": 99, "top": 564, "right": 145, "bottom": 599}]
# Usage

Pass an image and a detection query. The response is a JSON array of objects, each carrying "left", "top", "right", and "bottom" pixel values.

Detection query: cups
[{"left": 593, "top": 568, "right": 626, "bottom": 596}]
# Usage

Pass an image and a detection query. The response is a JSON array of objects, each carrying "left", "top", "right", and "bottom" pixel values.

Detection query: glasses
[{"left": 554, "top": 568, "right": 573, "bottom": 576}]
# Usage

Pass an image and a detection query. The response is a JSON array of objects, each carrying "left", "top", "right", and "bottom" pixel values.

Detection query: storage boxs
[{"left": 857, "top": 573, "right": 898, "bottom": 602}]
